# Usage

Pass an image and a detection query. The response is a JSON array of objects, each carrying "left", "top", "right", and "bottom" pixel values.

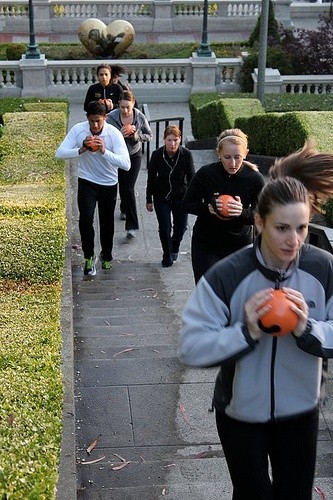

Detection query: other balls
[
  {"left": 258, "top": 290, "right": 299, "bottom": 335},
  {"left": 124, "top": 125, "right": 133, "bottom": 137},
  {"left": 86, "top": 137, "right": 100, "bottom": 151},
  {"left": 100, "top": 99, "right": 110, "bottom": 111},
  {"left": 215, "top": 195, "right": 235, "bottom": 219}
]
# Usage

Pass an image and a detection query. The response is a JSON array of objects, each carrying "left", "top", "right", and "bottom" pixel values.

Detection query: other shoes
[
  {"left": 120, "top": 212, "right": 127, "bottom": 220},
  {"left": 127, "top": 229, "right": 137, "bottom": 239},
  {"left": 163, "top": 251, "right": 178, "bottom": 267}
]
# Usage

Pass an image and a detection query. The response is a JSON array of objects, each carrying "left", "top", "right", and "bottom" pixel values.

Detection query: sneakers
[
  {"left": 84, "top": 255, "right": 99, "bottom": 276},
  {"left": 100, "top": 255, "right": 113, "bottom": 271}
]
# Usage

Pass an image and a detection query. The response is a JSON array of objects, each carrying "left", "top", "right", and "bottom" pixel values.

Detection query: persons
[
  {"left": 104, "top": 89, "right": 153, "bottom": 238},
  {"left": 108, "top": 67, "right": 138, "bottom": 109},
  {"left": 83, "top": 64, "right": 123, "bottom": 116},
  {"left": 146, "top": 125, "right": 195, "bottom": 267},
  {"left": 55, "top": 101, "right": 131, "bottom": 280},
  {"left": 176, "top": 128, "right": 333, "bottom": 500}
]
